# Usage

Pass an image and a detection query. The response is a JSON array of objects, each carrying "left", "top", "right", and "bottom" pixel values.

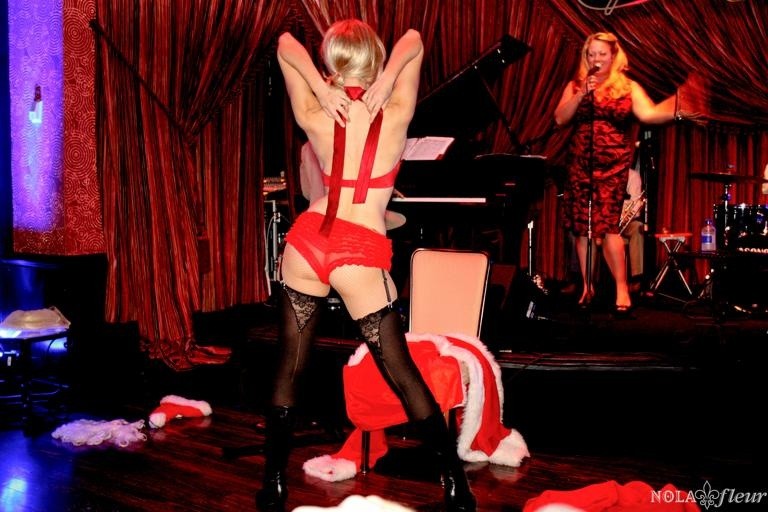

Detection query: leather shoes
[
  {"left": 562, "top": 283, "right": 579, "bottom": 294},
  {"left": 635, "top": 288, "right": 656, "bottom": 301}
]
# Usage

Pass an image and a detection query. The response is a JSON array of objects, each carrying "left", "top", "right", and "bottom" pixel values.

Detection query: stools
[{"left": 641, "top": 232, "right": 700, "bottom": 304}]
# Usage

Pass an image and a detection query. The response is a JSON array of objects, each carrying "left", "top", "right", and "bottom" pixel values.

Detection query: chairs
[
  {"left": 0, "top": 258, "right": 74, "bottom": 417},
  {"left": 359, "top": 246, "right": 492, "bottom": 474}
]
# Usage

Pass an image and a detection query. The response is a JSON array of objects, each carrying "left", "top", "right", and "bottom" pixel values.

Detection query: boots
[
  {"left": 254, "top": 405, "right": 296, "bottom": 511},
  {"left": 412, "top": 409, "right": 478, "bottom": 512}
]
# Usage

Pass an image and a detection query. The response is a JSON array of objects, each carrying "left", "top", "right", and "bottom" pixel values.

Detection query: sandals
[
  {"left": 615, "top": 297, "right": 634, "bottom": 315},
  {"left": 578, "top": 296, "right": 590, "bottom": 308}
]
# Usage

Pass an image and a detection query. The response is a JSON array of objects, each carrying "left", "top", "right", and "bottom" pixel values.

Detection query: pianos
[{"left": 374, "top": 33, "right": 558, "bottom": 249}]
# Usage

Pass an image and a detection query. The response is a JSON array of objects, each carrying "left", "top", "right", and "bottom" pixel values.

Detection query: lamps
[{"left": 29, "top": 86, "right": 44, "bottom": 125}]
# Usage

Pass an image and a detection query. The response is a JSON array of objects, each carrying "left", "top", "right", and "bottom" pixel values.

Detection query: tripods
[{"left": 545, "top": 90, "right": 629, "bottom": 341}]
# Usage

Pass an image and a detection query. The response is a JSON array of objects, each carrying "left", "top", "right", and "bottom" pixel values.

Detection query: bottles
[{"left": 701, "top": 220, "right": 715, "bottom": 251}]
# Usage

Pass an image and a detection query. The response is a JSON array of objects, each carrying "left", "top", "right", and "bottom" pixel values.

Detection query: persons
[
  {"left": 621, "top": 168, "right": 646, "bottom": 296},
  {"left": 255, "top": 16, "right": 476, "bottom": 512},
  {"left": 553, "top": 31, "right": 701, "bottom": 320}
]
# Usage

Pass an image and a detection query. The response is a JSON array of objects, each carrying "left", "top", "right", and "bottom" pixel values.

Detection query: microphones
[{"left": 585, "top": 63, "right": 601, "bottom": 78}]
